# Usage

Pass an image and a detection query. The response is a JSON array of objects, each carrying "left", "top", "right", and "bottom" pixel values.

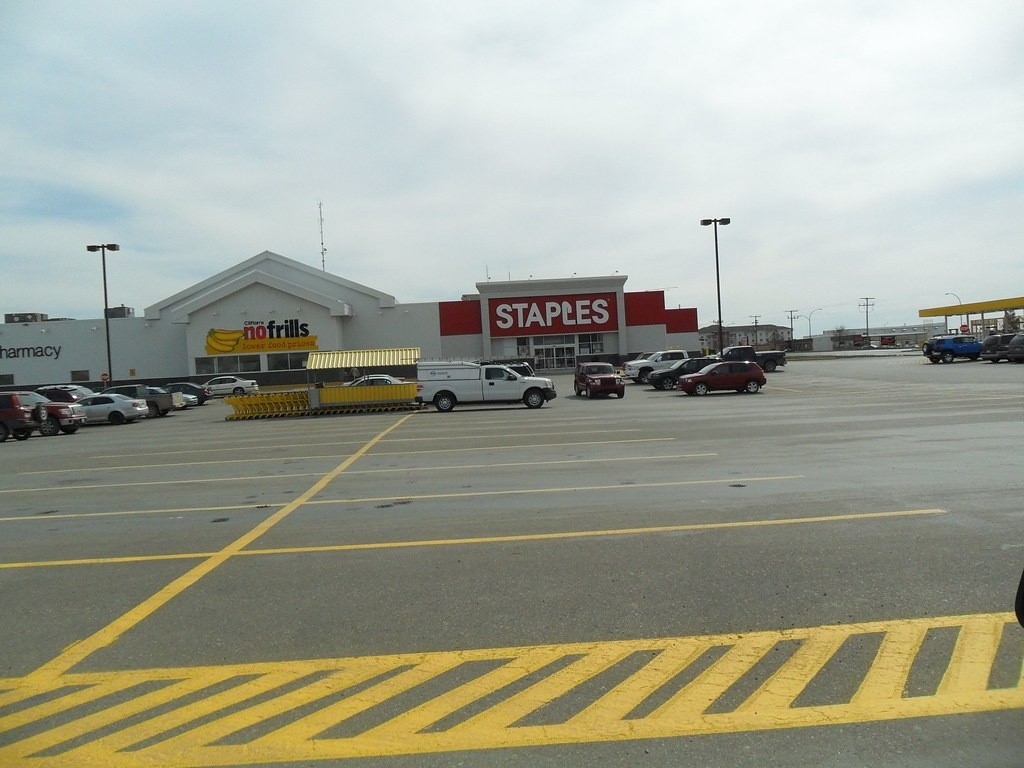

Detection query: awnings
[{"left": 307, "top": 348, "right": 420, "bottom": 369}]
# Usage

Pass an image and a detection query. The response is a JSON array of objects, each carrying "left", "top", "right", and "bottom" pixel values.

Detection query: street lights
[
  {"left": 798, "top": 308, "right": 822, "bottom": 339},
  {"left": 945, "top": 293, "right": 962, "bottom": 326},
  {"left": 701, "top": 217, "right": 730, "bottom": 360},
  {"left": 87, "top": 244, "right": 120, "bottom": 388}
]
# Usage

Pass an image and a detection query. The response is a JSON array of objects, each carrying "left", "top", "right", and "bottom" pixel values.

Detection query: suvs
[
  {"left": 34, "top": 385, "right": 93, "bottom": 404},
  {"left": 982, "top": 334, "right": 1024, "bottom": 363},
  {"left": 923, "top": 334, "right": 984, "bottom": 363},
  {"left": 676, "top": 361, "right": 766, "bottom": 395},
  {"left": 647, "top": 358, "right": 725, "bottom": 390},
  {"left": 574, "top": 362, "right": 625, "bottom": 397},
  {"left": 0, "top": 391, "right": 86, "bottom": 441},
  {"left": 499, "top": 362, "right": 535, "bottom": 378}
]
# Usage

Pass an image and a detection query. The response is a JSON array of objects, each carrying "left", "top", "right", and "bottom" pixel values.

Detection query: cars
[
  {"left": 343, "top": 374, "right": 416, "bottom": 386},
  {"left": 201, "top": 376, "right": 260, "bottom": 397},
  {"left": 76, "top": 392, "right": 149, "bottom": 424},
  {"left": 164, "top": 383, "right": 214, "bottom": 410}
]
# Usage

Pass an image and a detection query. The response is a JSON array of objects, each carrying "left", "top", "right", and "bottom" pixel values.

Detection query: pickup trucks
[
  {"left": 622, "top": 349, "right": 690, "bottom": 383},
  {"left": 97, "top": 385, "right": 172, "bottom": 418},
  {"left": 704, "top": 346, "right": 787, "bottom": 373},
  {"left": 415, "top": 361, "right": 557, "bottom": 411}
]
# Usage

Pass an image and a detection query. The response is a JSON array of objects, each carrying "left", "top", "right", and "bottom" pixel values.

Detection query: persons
[{"left": 350, "top": 367, "right": 360, "bottom": 380}]
